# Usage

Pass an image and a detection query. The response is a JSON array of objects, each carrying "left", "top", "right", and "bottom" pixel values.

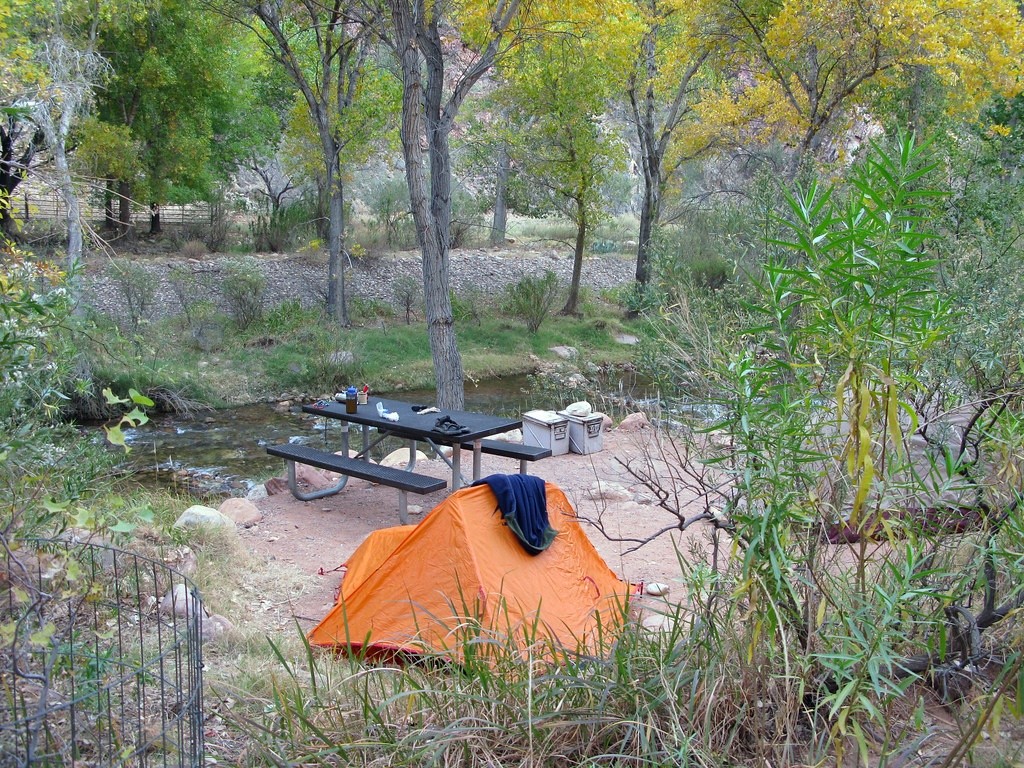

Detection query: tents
[{"left": 307, "top": 474, "right": 643, "bottom": 689}]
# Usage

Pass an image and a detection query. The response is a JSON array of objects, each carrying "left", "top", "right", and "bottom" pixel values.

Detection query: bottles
[{"left": 346, "top": 386, "right": 357, "bottom": 413}]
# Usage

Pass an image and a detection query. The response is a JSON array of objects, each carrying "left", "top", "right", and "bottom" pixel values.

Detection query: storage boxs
[
  {"left": 522, "top": 409, "right": 571, "bottom": 456},
  {"left": 556, "top": 409, "right": 604, "bottom": 456}
]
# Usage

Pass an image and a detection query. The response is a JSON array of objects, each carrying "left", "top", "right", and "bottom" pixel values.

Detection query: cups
[{"left": 357, "top": 391, "right": 367, "bottom": 404}]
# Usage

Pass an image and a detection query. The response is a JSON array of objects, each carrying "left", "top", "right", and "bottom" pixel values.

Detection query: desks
[{"left": 303, "top": 396, "right": 523, "bottom": 493}]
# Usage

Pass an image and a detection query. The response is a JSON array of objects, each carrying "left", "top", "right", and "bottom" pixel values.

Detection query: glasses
[{"left": 411, "top": 405, "right": 427, "bottom": 412}]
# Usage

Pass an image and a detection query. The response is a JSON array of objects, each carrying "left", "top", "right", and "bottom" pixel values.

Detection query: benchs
[
  {"left": 266, "top": 444, "right": 449, "bottom": 526},
  {"left": 379, "top": 428, "right": 553, "bottom": 481}
]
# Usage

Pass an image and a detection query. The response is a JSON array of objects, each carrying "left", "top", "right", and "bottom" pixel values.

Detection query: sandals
[{"left": 434, "top": 415, "right": 470, "bottom": 434}]
